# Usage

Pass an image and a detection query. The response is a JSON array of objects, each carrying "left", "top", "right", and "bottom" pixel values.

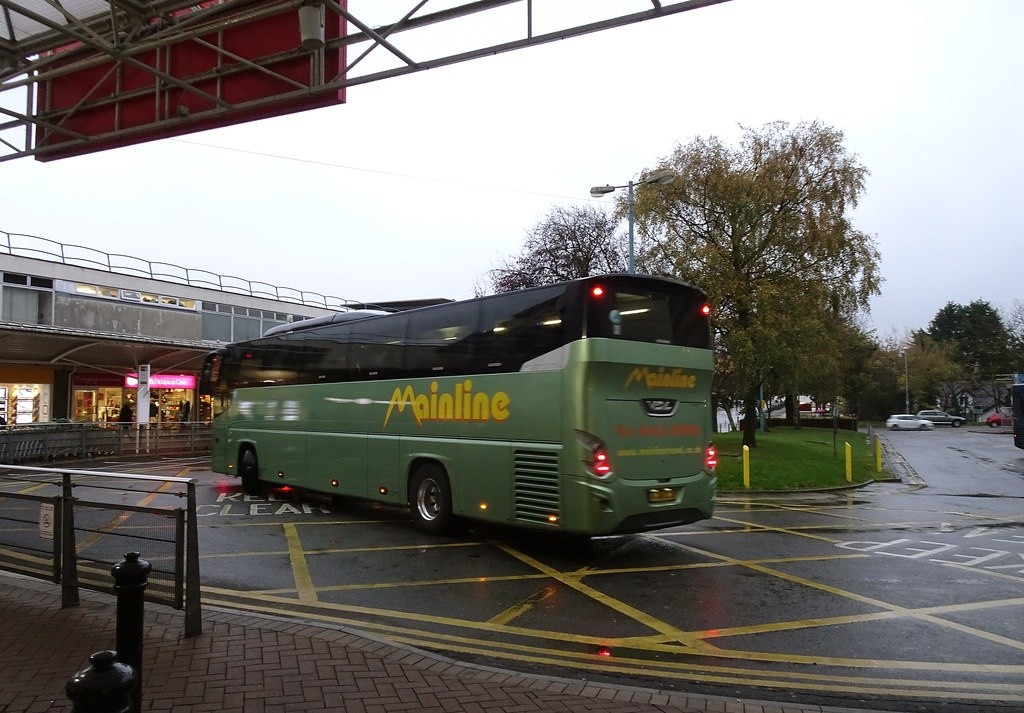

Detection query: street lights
[
  {"left": 899, "top": 351, "right": 909, "bottom": 415},
  {"left": 590, "top": 170, "right": 675, "bottom": 275}
]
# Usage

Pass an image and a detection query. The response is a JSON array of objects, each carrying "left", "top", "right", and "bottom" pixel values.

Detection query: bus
[
  {"left": 195, "top": 271, "right": 719, "bottom": 542},
  {"left": 1003, "top": 384, "right": 1024, "bottom": 451}
]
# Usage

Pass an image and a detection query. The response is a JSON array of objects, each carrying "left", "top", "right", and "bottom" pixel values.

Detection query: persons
[{"left": 117, "top": 400, "right": 133, "bottom": 426}]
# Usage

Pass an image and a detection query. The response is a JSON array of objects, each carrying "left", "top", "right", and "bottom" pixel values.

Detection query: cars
[
  {"left": 886, "top": 414, "right": 934, "bottom": 431},
  {"left": 986, "top": 412, "right": 1014, "bottom": 428}
]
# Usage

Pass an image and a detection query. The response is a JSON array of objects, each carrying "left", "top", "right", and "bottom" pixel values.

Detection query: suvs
[{"left": 916, "top": 410, "right": 966, "bottom": 428}]
